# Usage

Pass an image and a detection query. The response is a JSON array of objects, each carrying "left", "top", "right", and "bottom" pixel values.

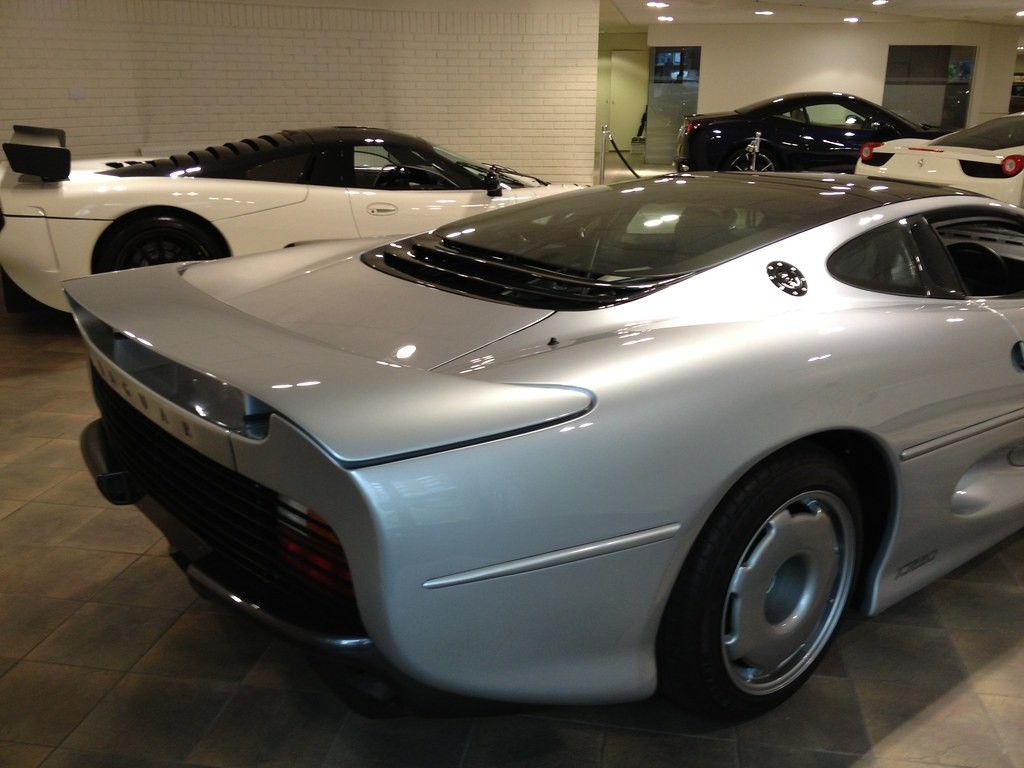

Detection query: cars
[
  {"left": 855, "top": 112, "right": 1023, "bottom": 203},
  {"left": 676, "top": 90, "right": 956, "bottom": 173}
]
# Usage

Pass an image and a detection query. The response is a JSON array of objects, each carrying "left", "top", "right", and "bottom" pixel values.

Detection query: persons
[{"left": 662, "top": 58, "right": 675, "bottom": 77}]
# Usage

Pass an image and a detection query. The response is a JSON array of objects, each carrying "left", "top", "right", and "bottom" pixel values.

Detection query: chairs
[{"left": 942, "top": 242, "right": 1013, "bottom": 298}]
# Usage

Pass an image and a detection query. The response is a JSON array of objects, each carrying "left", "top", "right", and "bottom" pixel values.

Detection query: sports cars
[
  {"left": 1, "top": 123, "right": 604, "bottom": 317},
  {"left": 65, "top": 173, "right": 1024, "bottom": 728}
]
